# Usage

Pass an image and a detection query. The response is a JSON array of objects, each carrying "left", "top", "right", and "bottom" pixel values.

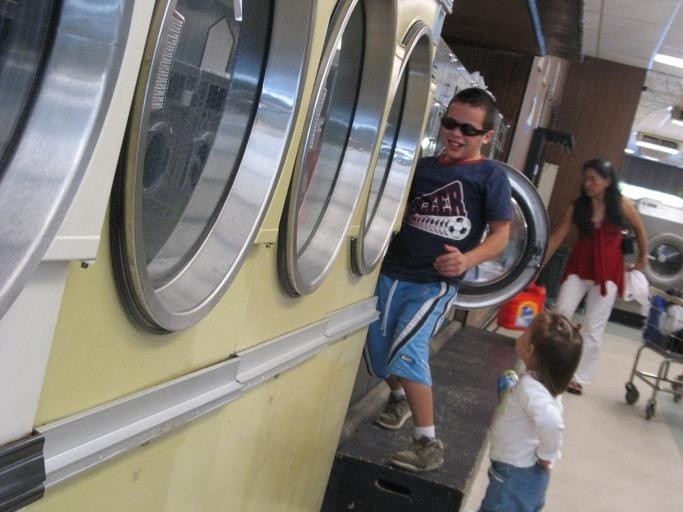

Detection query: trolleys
[{"left": 625, "top": 293, "right": 683, "bottom": 421}]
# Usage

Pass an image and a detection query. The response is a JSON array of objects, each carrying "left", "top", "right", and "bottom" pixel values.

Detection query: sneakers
[
  {"left": 566, "top": 382, "right": 582, "bottom": 395},
  {"left": 389, "top": 435, "right": 446, "bottom": 472},
  {"left": 374, "top": 393, "right": 411, "bottom": 430}
]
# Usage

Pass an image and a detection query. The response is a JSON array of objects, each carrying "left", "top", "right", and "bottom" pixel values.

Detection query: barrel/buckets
[{"left": 500, "top": 285, "right": 546, "bottom": 331}]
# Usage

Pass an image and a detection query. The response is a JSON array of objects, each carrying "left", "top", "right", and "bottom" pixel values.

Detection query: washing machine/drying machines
[
  {"left": 609, "top": 196, "right": 683, "bottom": 315},
  {"left": 0, "top": 0, "right": 550, "bottom": 512}
]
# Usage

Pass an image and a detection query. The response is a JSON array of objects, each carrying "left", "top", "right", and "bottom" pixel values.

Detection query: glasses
[{"left": 440, "top": 115, "right": 488, "bottom": 137}]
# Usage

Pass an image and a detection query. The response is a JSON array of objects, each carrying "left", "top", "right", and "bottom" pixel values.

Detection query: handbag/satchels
[{"left": 622, "top": 233, "right": 635, "bottom": 254}]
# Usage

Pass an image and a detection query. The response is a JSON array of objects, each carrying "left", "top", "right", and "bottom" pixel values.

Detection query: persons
[
  {"left": 361, "top": 86, "right": 514, "bottom": 474},
  {"left": 530, "top": 155, "right": 649, "bottom": 396},
  {"left": 478, "top": 307, "right": 585, "bottom": 512}
]
